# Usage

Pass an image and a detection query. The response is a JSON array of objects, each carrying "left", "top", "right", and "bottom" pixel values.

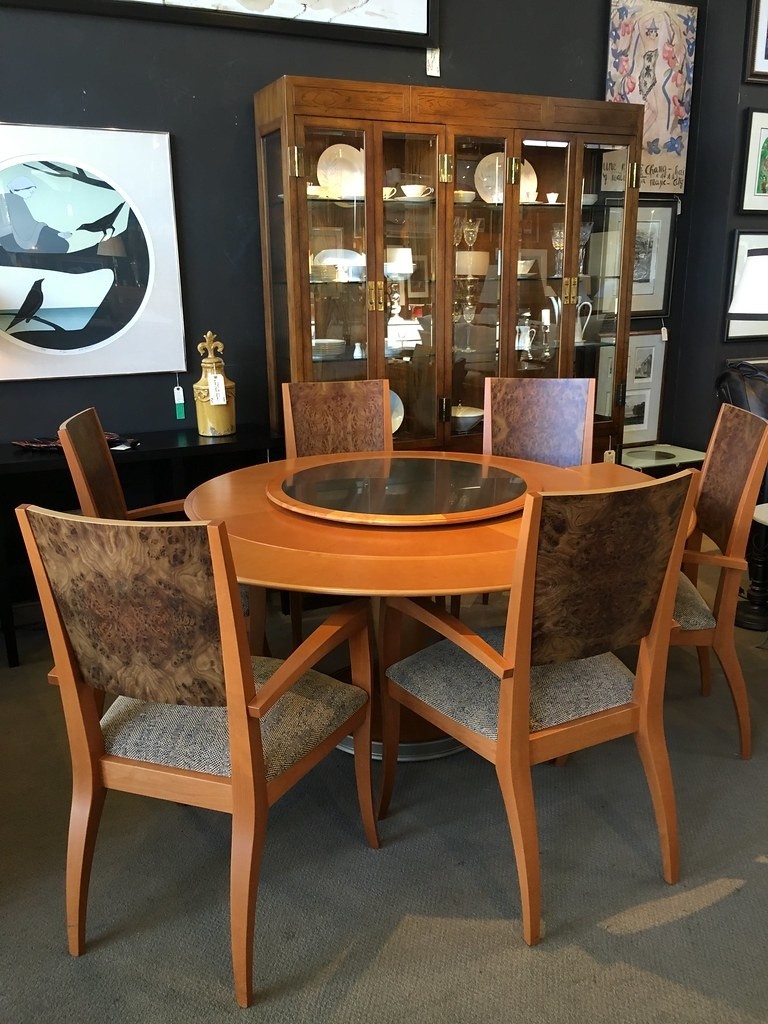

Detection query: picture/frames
[
  {"left": 726, "top": 228, "right": 768, "bottom": 316},
  {"left": 737, "top": 107, "right": 768, "bottom": 217},
  {"left": 741, "top": 0, "right": 768, "bottom": 85},
  {"left": 595, "top": 0, "right": 699, "bottom": 194},
  {"left": 595, "top": 199, "right": 679, "bottom": 326},
  {"left": 595, "top": 329, "right": 667, "bottom": 447},
  {"left": 0, "top": 120, "right": 188, "bottom": 382},
  {"left": 723, "top": 317, "right": 768, "bottom": 342}
]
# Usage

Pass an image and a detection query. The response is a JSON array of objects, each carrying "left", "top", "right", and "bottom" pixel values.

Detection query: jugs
[
  {"left": 518, "top": 325, "right": 536, "bottom": 351},
  {"left": 548, "top": 296, "right": 592, "bottom": 343}
]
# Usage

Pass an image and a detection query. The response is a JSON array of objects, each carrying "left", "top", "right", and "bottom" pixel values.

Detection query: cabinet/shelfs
[{"left": 252, "top": 74, "right": 643, "bottom": 448}]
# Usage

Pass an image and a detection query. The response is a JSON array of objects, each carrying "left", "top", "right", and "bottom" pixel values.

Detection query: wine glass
[
  {"left": 453, "top": 216, "right": 463, "bottom": 280},
  {"left": 452, "top": 300, "right": 461, "bottom": 352},
  {"left": 580, "top": 222, "right": 595, "bottom": 276},
  {"left": 459, "top": 216, "right": 484, "bottom": 279},
  {"left": 462, "top": 301, "right": 476, "bottom": 352},
  {"left": 549, "top": 222, "right": 565, "bottom": 278}
]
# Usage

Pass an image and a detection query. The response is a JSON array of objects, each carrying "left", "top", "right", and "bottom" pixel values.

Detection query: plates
[
  {"left": 390, "top": 389, "right": 404, "bottom": 433},
  {"left": 311, "top": 339, "right": 346, "bottom": 356},
  {"left": 395, "top": 196, "right": 432, "bottom": 201},
  {"left": 317, "top": 144, "right": 365, "bottom": 207},
  {"left": 474, "top": 152, "right": 537, "bottom": 203},
  {"left": 384, "top": 199, "right": 394, "bottom": 200},
  {"left": 516, "top": 273, "right": 537, "bottom": 278}
]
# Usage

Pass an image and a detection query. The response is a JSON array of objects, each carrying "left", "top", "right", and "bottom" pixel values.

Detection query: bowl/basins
[
  {"left": 450, "top": 407, "right": 485, "bottom": 434},
  {"left": 384, "top": 263, "right": 417, "bottom": 280},
  {"left": 454, "top": 191, "right": 476, "bottom": 203}
]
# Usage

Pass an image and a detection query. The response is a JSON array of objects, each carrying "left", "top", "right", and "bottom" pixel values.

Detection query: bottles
[
  {"left": 192, "top": 330, "right": 235, "bottom": 437},
  {"left": 353, "top": 343, "right": 362, "bottom": 358}
]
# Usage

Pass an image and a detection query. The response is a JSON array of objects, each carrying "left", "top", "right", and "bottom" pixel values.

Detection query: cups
[
  {"left": 546, "top": 193, "right": 559, "bottom": 202},
  {"left": 401, "top": 185, "right": 435, "bottom": 197},
  {"left": 311, "top": 265, "right": 337, "bottom": 281},
  {"left": 518, "top": 259, "right": 536, "bottom": 273},
  {"left": 345, "top": 265, "right": 366, "bottom": 282},
  {"left": 582, "top": 194, "right": 598, "bottom": 205},
  {"left": 383, "top": 187, "right": 396, "bottom": 199}
]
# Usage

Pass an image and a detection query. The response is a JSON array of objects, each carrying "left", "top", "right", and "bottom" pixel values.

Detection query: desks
[
  {"left": 0, "top": 427, "right": 272, "bottom": 668},
  {"left": 183, "top": 451, "right": 662, "bottom": 762}
]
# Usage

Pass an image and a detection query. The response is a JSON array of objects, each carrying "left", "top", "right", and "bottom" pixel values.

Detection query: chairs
[{"left": 0, "top": 375, "right": 768, "bottom": 1007}]
